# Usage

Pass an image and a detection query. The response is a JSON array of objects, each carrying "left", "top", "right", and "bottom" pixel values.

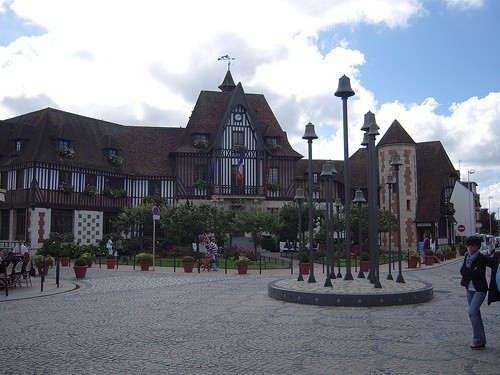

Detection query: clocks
[{"left": 234, "top": 112, "right": 243, "bottom": 122}]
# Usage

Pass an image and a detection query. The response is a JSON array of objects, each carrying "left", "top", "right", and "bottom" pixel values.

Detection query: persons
[
  {"left": 464, "top": 250, "right": 500, "bottom": 306},
  {"left": 116, "top": 236, "right": 123, "bottom": 258},
  {"left": 197, "top": 232, "right": 219, "bottom": 272},
  {"left": 281, "top": 236, "right": 320, "bottom": 256},
  {"left": 107, "top": 235, "right": 114, "bottom": 255},
  {"left": 0, "top": 239, "right": 31, "bottom": 282},
  {"left": 458, "top": 236, "right": 488, "bottom": 348},
  {"left": 420, "top": 233, "right": 435, "bottom": 264}
]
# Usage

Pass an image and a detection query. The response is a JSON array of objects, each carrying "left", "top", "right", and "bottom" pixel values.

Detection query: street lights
[
  {"left": 489, "top": 196, "right": 497, "bottom": 237},
  {"left": 211, "top": 196, "right": 220, "bottom": 239},
  {"left": 468, "top": 169, "right": 478, "bottom": 236},
  {"left": 295, "top": 73, "right": 406, "bottom": 290},
  {"left": 257, "top": 227, "right": 262, "bottom": 273}
]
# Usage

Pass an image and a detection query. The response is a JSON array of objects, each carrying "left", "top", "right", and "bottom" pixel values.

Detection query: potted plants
[
  {"left": 83, "top": 253, "right": 96, "bottom": 260},
  {"left": 73, "top": 258, "right": 88, "bottom": 278},
  {"left": 61, "top": 249, "right": 70, "bottom": 266},
  {"left": 460, "top": 243, "right": 467, "bottom": 255},
  {"left": 425, "top": 249, "right": 434, "bottom": 265},
  {"left": 105, "top": 254, "right": 116, "bottom": 269},
  {"left": 436, "top": 243, "right": 457, "bottom": 261},
  {"left": 182, "top": 256, "right": 195, "bottom": 272},
  {"left": 299, "top": 252, "right": 311, "bottom": 275},
  {"left": 361, "top": 252, "right": 370, "bottom": 272}
]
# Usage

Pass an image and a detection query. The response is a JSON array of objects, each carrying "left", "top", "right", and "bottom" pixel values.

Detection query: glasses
[{"left": 466, "top": 243, "right": 476, "bottom": 247}]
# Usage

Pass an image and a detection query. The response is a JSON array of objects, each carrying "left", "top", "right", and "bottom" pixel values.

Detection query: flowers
[
  {"left": 403, "top": 250, "right": 420, "bottom": 261},
  {"left": 33, "top": 254, "right": 55, "bottom": 268},
  {"left": 55, "top": 147, "right": 125, "bottom": 198},
  {"left": 232, "top": 256, "right": 251, "bottom": 265},
  {"left": 136, "top": 253, "right": 154, "bottom": 261},
  {"left": 267, "top": 143, "right": 281, "bottom": 151},
  {"left": 193, "top": 137, "right": 209, "bottom": 149},
  {"left": 193, "top": 179, "right": 210, "bottom": 190}
]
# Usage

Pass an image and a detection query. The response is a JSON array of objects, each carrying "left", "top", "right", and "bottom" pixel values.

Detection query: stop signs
[{"left": 458, "top": 225, "right": 466, "bottom": 232}]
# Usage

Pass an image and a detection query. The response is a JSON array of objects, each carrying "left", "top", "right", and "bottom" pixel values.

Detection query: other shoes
[{"left": 470, "top": 341, "right": 487, "bottom": 348}]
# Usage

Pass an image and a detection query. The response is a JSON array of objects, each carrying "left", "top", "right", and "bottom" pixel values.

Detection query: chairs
[{"left": 0, "top": 260, "right": 32, "bottom": 296}]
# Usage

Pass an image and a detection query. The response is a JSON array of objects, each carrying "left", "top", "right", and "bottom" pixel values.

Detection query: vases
[
  {"left": 87, "top": 261, "right": 93, "bottom": 268},
  {"left": 37, "top": 265, "right": 48, "bottom": 275},
  {"left": 407, "top": 257, "right": 417, "bottom": 268},
  {"left": 140, "top": 260, "right": 150, "bottom": 271},
  {"left": 238, "top": 263, "right": 247, "bottom": 274}
]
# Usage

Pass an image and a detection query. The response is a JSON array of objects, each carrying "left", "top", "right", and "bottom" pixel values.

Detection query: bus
[{"left": 475, "top": 234, "right": 496, "bottom": 256}]
